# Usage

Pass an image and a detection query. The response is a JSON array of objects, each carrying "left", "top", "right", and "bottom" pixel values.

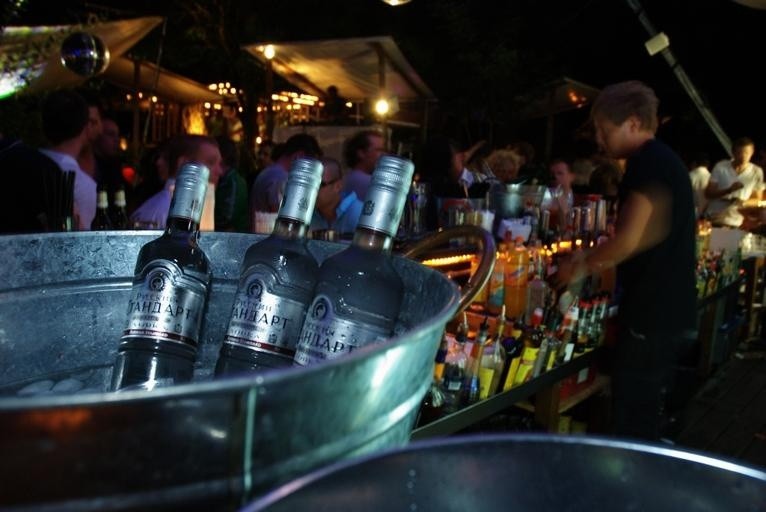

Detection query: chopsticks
[{"left": 40, "top": 170, "right": 76, "bottom": 217}]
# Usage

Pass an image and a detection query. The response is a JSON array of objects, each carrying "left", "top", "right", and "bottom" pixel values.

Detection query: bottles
[
  {"left": 90, "top": 179, "right": 111, "bottom": 229},
  {"left": 110, "top": 183, "right": 129, "bottom": 229},
  {"left": 215, "top": 158, "right": 324, "bottom": 379},
  {"left": 293, "top": 154, "right": 414, "bottom": 369},
  {"left": 109, "top": 162, "right": 213, "bottom": 389},
  {"left": 695, "top": 245, "right": 743, "bottom": 299},
  {"left": 418, "top": 176, "right": 611, "bottom": 426}
]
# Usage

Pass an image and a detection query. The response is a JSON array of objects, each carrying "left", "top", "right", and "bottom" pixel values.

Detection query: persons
[
  {"left": 0, "top": 88, "right": 132, "bottom": 240},
  {"left": 134, "top": 131, "right": 386, "bottom": 241},
  {"left": 410, "top": 140, "right": 623, "bottom": 235},
  {"left": 556, "top": 81, "right": 702, "bottom": 449},
  {"left": 688, "top": 139, "right": 766, "bottom": 222}
]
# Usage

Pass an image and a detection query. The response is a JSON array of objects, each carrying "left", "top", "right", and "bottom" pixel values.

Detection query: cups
[
  {"left": 132, "top": 221, "right": 159, "bottom": 230},
  {"left": 36, "top": 213, "right": 79, "bottom": 232}
]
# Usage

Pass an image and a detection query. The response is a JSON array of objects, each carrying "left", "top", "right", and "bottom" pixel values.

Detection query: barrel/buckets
[
  {"left": 1, "top": 226, "right": 498, "bottom": 512},
  {"left": 246, "top": 434, "right": 764, "bottom": 511}
]
